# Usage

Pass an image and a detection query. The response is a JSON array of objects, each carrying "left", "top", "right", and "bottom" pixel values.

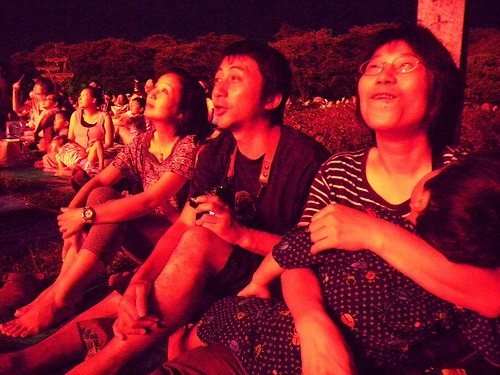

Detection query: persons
[
  {"left": 0, "top": 41, "right": 332, "bottom": 375},
  {"left": 167, "top": 160, "right": 500, "bottom": 375},
  {"left": 13, "top": 76, "right": 213, "bottom": 194},
  {"left": 0, "top": 68, "right": 210, "bottom": 339},
  {"left": 150, "top": 20, "right": 500, "bottom": 375}
]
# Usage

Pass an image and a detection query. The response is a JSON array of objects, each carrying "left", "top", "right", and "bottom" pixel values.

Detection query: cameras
[{"left": 189, "top": 184, "right": 227, "bottom": 220}]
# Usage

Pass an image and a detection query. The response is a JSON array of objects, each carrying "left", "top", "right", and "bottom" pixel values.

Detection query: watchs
[{"left": 81, "top": 205, "right": 95, "bottom": 224}]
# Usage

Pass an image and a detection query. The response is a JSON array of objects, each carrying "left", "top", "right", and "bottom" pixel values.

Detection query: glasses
[{"left": 358, "top": 55, "right": 431, "bottom": 76}]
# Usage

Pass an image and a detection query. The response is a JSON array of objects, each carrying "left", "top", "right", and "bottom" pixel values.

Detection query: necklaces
[{"left": 156, "top": 136, "right": 175, "bottom": 162}]
